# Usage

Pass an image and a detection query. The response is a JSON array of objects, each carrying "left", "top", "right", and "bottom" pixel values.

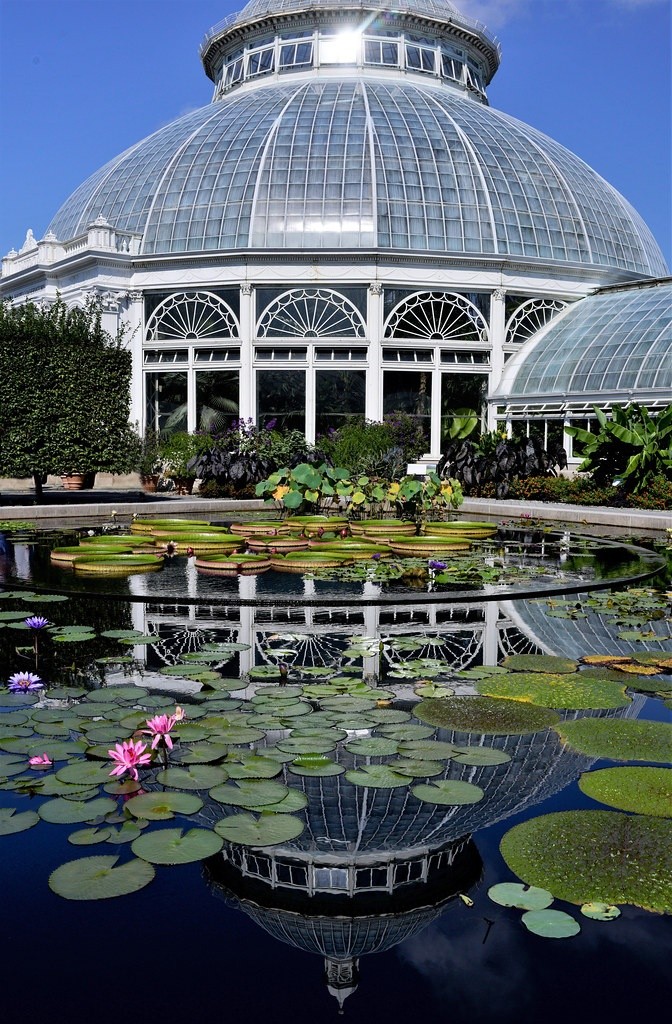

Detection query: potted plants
[
  {"left": 151, "top": 431, "right": 215, "bottom": 495},
  {"left": 139, "top": 426, "right": 163, "bottom": 492}
]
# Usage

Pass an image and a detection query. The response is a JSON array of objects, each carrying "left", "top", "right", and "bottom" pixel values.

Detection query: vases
[
  {"left": 60, "top": 476, "right": 69, "bottom": 489},
  {"left": 64, "top": 473, "right": 84, "bottom": 490}
]
